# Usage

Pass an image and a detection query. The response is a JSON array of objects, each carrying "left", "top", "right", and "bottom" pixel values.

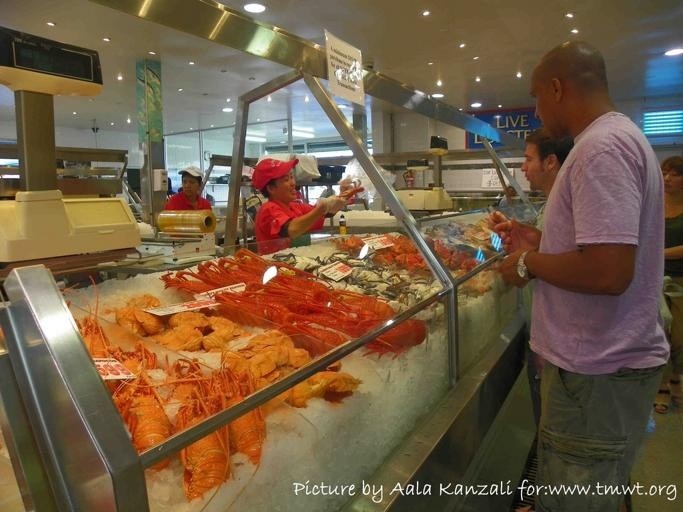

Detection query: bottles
[
  {"left": 404, "top": 172, "right": 414, "bottom": 188},
  {"left": 338, "top": 214, "right": 347, "bottom": 234}
]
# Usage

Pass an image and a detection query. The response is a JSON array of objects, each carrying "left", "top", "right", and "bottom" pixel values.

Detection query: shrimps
[
  {"left": 63, "top": 276, "right": 268, "bottom": 503},
  {"left": 159, "top": 247, "right": 427, "bottom": 360}
]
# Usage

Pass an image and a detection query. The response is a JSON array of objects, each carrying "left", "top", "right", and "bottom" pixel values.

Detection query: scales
[{"left": 386, "top": 135, "right": 453, "bottom": 211}]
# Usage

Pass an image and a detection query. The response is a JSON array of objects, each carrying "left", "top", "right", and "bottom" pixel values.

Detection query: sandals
[
  {"left": 654, "top": 386, "right": 670, "bottom": 414},
  {"left": 668, "top": 381, "right": 683, "bottom": 406}
]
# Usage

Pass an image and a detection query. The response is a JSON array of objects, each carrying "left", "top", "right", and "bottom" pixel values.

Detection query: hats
[
  {"left": 180, "top": 166, "right": 203, "bottom": 178},
  {"left": 253, "top": 159, "right": 298, "bottom": 190}
]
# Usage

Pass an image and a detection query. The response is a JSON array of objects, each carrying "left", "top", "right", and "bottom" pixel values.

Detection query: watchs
[{"left": 517, "top": 251, "right": 529, "bottom": 282}]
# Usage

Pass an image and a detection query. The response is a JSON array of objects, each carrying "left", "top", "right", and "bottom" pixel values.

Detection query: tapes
[{"left": 157, "top": 209, "right": 216, "bottom": 233}]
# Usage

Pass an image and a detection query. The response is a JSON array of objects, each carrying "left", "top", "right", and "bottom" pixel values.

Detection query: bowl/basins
[{"left": 311, "top": 164, "right": 345, "bottom": 184}]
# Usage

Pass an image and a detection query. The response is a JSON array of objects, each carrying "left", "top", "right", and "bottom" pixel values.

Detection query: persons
[
  {"left": 250, "top": 156, "right": 346, "bottom": 255},
  {"left": 167, "top": 177, "right": 176, "bottom": 200},
  {"left": 165, "top": 165, "right": 212, "bottom": 210},
  {"left": 498, "top": 186, "right": 516, "bottom": 206},
  {"left": 500, "top": 39, "right": 670, "bottom": 512},
  {"left": 652, "top": 155, "right": 683, "bottom": 414},
  {"left": 490, "top": 128, "right": 572, "bottom": 427}
]
